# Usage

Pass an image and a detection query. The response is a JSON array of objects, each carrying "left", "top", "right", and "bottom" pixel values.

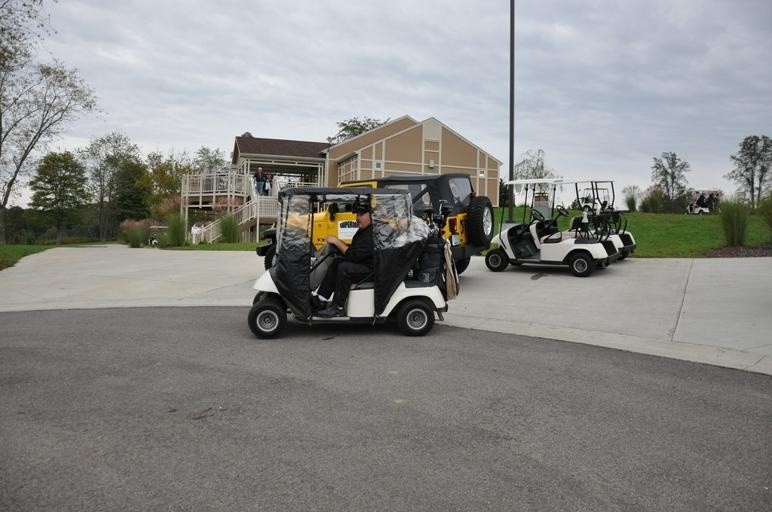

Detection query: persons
[
  {"left": 696, "top": 192, "right": 720, "bottom": 209},
  {"left": 309, "top": 201, "right": 377, "bottom": 317},
  {"left": 253, "top": 167, "right": 270, "bottom": 196}
]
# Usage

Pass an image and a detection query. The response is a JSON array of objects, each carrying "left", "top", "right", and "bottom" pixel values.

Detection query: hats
[{"left": 352, "top": 204, "right": 371, "bottom": 214}]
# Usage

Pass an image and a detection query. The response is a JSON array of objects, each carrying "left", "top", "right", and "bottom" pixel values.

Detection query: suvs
[{"left": 253, "top": 172, "right": 495, "bottom": 282}]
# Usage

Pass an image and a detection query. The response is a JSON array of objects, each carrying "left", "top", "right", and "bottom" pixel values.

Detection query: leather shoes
[
  {"left": 310, "top": 296, "right": 327, "bottom": 310},
  {"left": 317, "top": 303, "right": 347, "bottom": 318}
]
